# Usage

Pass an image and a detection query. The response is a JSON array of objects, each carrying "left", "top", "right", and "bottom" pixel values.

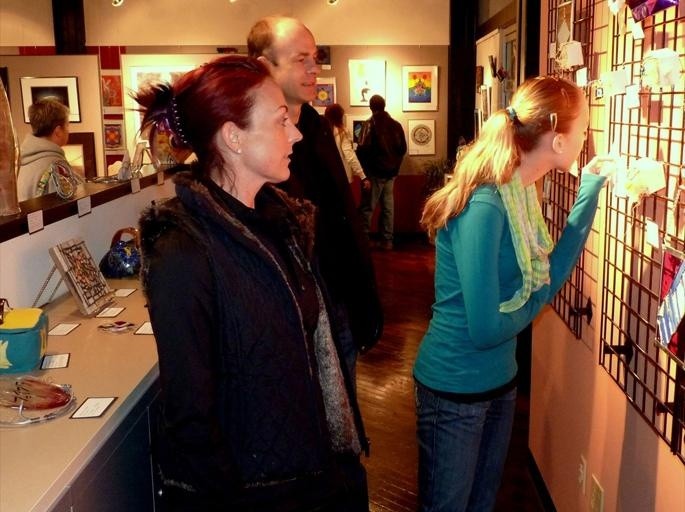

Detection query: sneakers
[{"left": 364, "top": 235, "right": 395, "bottom": 252}]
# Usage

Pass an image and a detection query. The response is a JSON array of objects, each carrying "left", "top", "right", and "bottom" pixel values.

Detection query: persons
[
  {"left": 16, "top": 99, "right": 83, "bottom": 203},
  {"left": 356, "top": 94, "right": 408, "bottom": 250},
  {"left": 242, "top": 14, "right": 383, "bottom": 359},
  {"left": 412, "top": 74, "right": 618, "bottom": 512},
  {"left": 130, "top": 53, "right": 370, "bottom": 511},
  {"left": 324, "top": 103, "right": 372, "bottom": 192}
]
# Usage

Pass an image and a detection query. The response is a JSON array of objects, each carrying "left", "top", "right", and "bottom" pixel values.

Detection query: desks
[{"left": 1, "top": 255, "right": 168, "bottom": 512}]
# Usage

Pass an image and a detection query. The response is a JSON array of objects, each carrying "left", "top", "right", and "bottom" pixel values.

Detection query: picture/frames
[
  {"left": 401, "top": 64, "right": 440, "bottom": 112},
  {"left": 344, "top": 113, "right": 373, "bottom": 153},
  {"left": 407, "top": 118, "right": 437, "bottom": 156},
  {"left": 49, "top": 236, "right": 116, "bottom": 316},
  {"left": 57, "top": 131, "right": 97, "bottom": 180},
  {"left": 17, "top": 75, "right": 82, "bottom": 124}
]
